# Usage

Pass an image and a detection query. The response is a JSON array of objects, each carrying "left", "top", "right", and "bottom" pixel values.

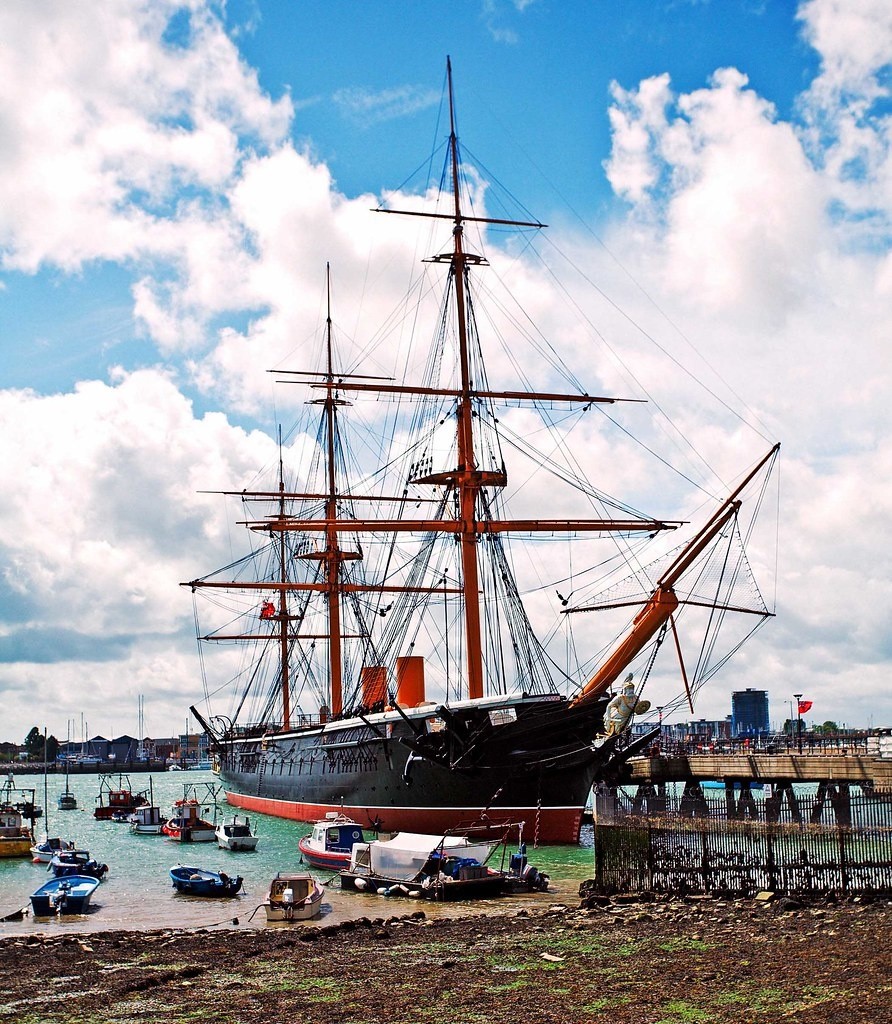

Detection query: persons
[
  {"left": 650, "top": 743, "right": 661, "bottom": 756},
  {"left": 602, "top": 680, "right": 640, "bottom": 737}
]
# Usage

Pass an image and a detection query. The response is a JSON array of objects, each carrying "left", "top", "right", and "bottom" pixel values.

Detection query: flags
[
  {"left": 799, "top": 701, "right": 812, "bottom": 713},
  {"left": 262, "top": 602, "right": 275, "bottom": 616}
]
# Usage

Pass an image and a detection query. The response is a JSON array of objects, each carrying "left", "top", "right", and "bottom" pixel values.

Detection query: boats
[
  {"left": 0, "top": 774, "right": 43, "bottom": 858},
  {"left": 163, "top": 781, "right": 219, "bottom": 844},
  {"left": 93, "top": 772, "right": 150, "bottom": 823},
  {"left": 297, "top": 796, "right": 369, "bottom": 874},
  {"left": 264, "top": 872, "right": 324, "bottom": 922},
  {"left": 168, "top": 863, "right": 244, "bottom": 899},
  {"left": 214, "top": 813, "right": 259, "bottom": 849},
  {"left": 338, "top": 834, "right": 512, "bottom": 902},
  {"left": 30, "top": 838, "right": 109, "bottom": 881},
  {"left": 28, "top": 874, "right": 101, "bottom": 916}
]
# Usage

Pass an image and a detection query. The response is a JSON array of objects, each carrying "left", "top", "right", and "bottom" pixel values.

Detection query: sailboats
[
  {"left": 178, "top": 50, "right": 785, "bottom": 849},
  {"left": 127, "top": 774, "right": 169, "bottom": 835},
  {"left": 57, "top": 720, "right": 78, "bottom": 811}
]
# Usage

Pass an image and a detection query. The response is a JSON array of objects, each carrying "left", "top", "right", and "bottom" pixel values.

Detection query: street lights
[
  {"left": 793, "top": 693, "right": 803, "bottom": 754},
  {"left": 655, "top": 706, "right": 664, "bottom": 742}
]
